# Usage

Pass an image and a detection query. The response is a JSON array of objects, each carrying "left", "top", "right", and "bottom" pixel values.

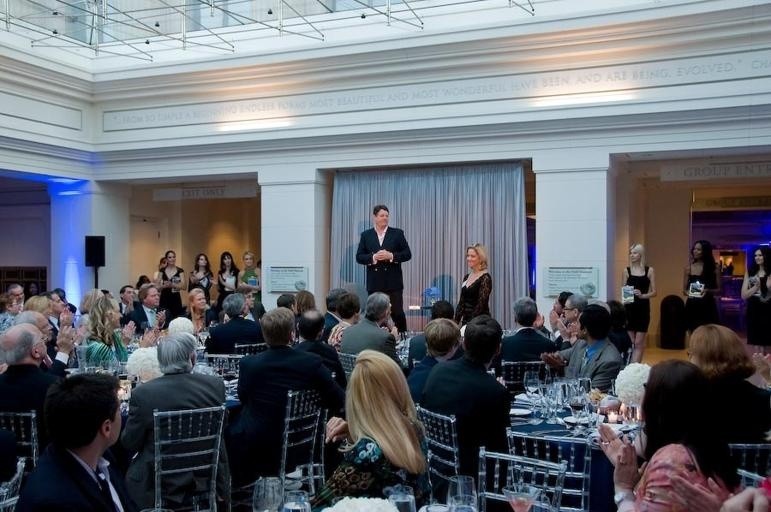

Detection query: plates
[
  {"left": 509, "top": 409, "right": 531, "bottom": 416},
  {"left": 620, "top": 424, "right": 639, "bottom": 431},
  {"left": 426, "top": 505, "right": 447, "bottom": 512}
]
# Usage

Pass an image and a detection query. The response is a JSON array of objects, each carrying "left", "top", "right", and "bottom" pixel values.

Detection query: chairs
[
  {"left": 479, "top": 428, "right": 592, "bottom": 512},
  {"left": 0, "top": 408, "right": 39, "bottom": 512},
  {"left": 151, "top": 402, "right": 226, "bottom": 512},
  {"left": 196, "top": 344, "right": 269, "bottom": 382},
  {"left": 487, "top": 359, "right": 545, "bottom": 397},
  {"left": 232, "top": 389, "right": 328, "bottom": 512},
  {"left": 414, "top": 403, "right": 462, "bottom": 508},
  {"left": 336, "top": 351, "right": 358, "bottom": 381},
  {"left": 728, "top": 443, "right": 771, "bottom": 487}
]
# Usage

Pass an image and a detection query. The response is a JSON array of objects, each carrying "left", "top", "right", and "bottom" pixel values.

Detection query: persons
[{"left": 357, "top": 206, "right": 412, "bottom": 331}]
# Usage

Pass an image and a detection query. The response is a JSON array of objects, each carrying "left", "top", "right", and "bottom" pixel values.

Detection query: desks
[
  {"left": 394, "top": 331, "right": 425, "bottom": 368},
  {"left": 65, "top": 380, "right": 260, "bottom": 455},
  {"left": 508, "top": 383, "right": 646, "bottom": 512}
]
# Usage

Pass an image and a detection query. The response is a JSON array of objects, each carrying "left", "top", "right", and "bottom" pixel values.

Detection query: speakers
[{"left": 85, "top": 235, "right": 105, "bottom": 267}]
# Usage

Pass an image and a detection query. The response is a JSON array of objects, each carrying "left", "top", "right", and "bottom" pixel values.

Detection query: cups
[
  {"left": 389, "top": 486, "right": 417, "bottom": 512},
  {"left": 446, "top": 476, "right": 479, "bottom": 512},
  {"left": 502, "top": 486, "right": 541, "bottom": 512},
  {"left": 252, "top": 477, "right": 311, "bottom": 512}
]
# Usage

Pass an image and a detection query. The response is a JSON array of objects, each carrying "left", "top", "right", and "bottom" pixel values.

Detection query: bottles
[
  {"left": 194, "top": 364, "right": 219, "bottom": 378},
  {"left": 79, "top": 359, "right": 140, "bottom": 408},
  {"left": 430, "top": 296, "right": 441, "bottom": 306},
  {"left": 607, "top": 406, "right": 636, "bottom": 424}
]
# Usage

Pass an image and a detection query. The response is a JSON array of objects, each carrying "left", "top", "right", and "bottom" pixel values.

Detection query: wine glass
[
  {"left": 199, "top": 332, "right": 208, "bottom": 349},
  {"left": 524, "top": 371, "right": 599, "bottom": 432},
  {"left": 222, "top": 362, "right": 238, "bottom": 400},
  {"left": 398, "top": 329, "right": 416, "bottom": 358}
]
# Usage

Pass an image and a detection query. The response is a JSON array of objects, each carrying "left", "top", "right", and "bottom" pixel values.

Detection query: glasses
[
  {"left": 145, "top": 291, "right": 161, "bottom": 297},
  {"left": 643, "top": 382, "right": 649, "bottom": 389},
  {"left": 346, "top": 372, "right": 353, "bottom": 385},
  {"left": 562, "top": 306, "right": 576, "bottom": 312},
  {"left": 686, "top": 350, "right": 693, "bottom": 357}
]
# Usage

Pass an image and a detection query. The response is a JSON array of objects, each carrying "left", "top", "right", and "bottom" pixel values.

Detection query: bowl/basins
[{"left": 600, "top": 405, "right": 619, "bottom": 414}]
